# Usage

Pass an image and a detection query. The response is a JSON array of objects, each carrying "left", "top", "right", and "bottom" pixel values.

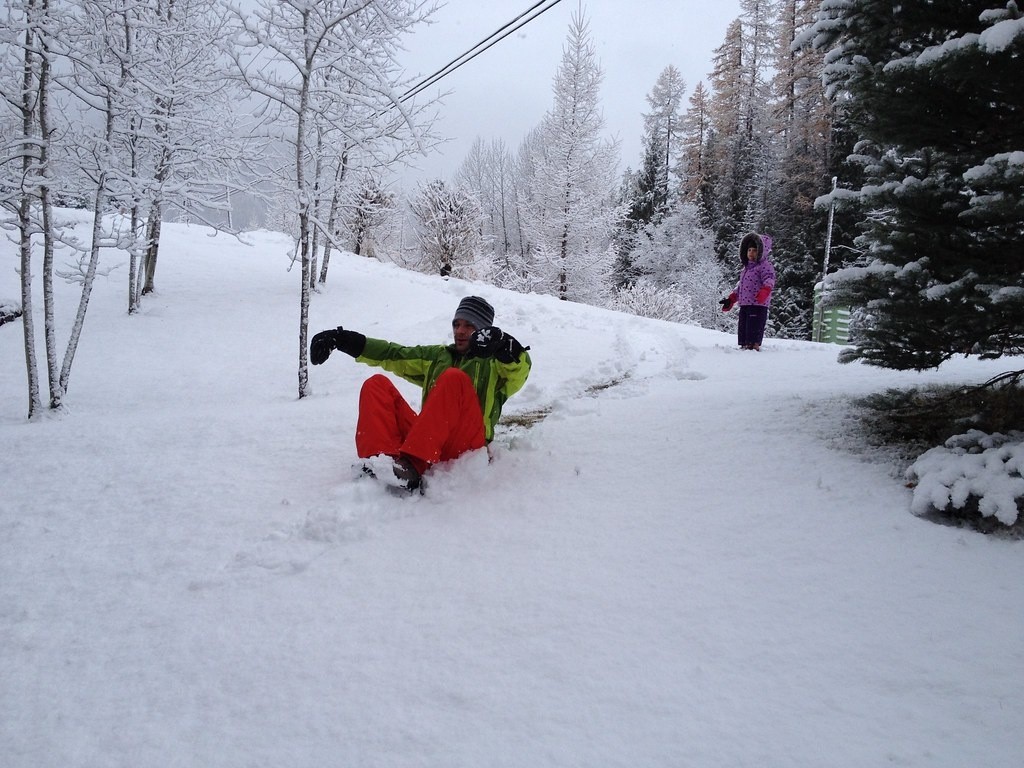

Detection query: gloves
[
  {"left": 755, "top": 286, "right": 771, "bottom": 304},
  {"left": 310, "top": 326, "right": 366, "bottom": 365},
  {"left": 469, "top": 326, "right": 530, "bottom": 364},
  {"left": 719, "top": 291, "right": 737, "bottom": 312}
]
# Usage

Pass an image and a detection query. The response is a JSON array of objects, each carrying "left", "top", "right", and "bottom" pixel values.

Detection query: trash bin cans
[{"left": 812, "top": 282, "right": 852, "bottom": 346}]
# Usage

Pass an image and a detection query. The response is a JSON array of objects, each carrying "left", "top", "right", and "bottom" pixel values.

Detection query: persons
[
  {"left": 719, "top": 233, "right": 776, "bottom": 350},
  {"left": 310, "top": 296, "right": 532, "bottom": 497}
]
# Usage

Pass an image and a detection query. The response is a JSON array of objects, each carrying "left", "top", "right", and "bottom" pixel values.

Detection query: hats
[{"left": 452, "top": 296, "right": 494, "bottom": 331}]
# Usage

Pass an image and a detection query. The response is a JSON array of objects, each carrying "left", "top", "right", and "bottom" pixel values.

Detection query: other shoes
[
  {"left": 352, "top": 455, "right": 427, "bottom": 496},
  {"left": 742, "top": 343, "right": 759, "bottom": 351}
]
